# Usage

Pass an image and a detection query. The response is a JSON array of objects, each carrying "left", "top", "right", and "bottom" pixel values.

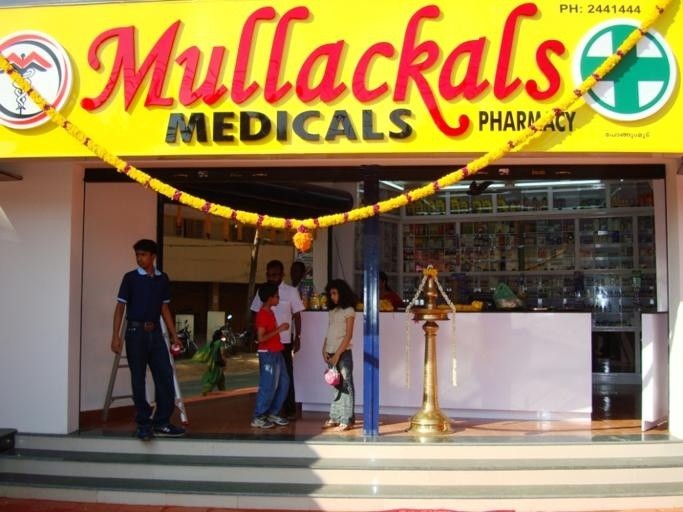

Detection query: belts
[{"left": 126, "top": 320, "right": 160, "bottom": 329}]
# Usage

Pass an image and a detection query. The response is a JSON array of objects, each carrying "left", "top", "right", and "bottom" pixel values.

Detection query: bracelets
[
  {"left": 295, "top": 334, "right": 301, "bottom": 339},
  {"left": 169, "top": 333, "right": 177, "bottom": 342}
]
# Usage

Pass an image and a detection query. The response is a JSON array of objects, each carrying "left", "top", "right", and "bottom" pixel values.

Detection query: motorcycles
[
  {"left": 170, "top": 320, "right": 199, "bottom": 361},
  {"left": 212, "top": 314, "right": 248, "bottom": 361}
]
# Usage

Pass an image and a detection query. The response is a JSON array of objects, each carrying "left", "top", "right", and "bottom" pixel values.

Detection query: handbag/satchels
[{"left": 322, "top": 359, "right": 341, "bottom": 386}]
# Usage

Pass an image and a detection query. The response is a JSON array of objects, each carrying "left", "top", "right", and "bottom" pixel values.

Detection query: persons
[
  {"left": 319, "top": 277, "right": 359, "bottom": 433},
  {"left": 107, "top": 237, "right": 188, "bottom": 443},
  {"left": 284, "top": 259, "right": 316, "bottom": 300},
  {"left": 360, "top": 270, "right": 404, "bottom": 310},
  {"left": 243, "top": 281, "right": 293, "bottom": 429},
  {"left": 247, "top": 258, "right": 307, "bottom": 422},
  {"left": 191, "top": 329, "right": 229, "bottom": 398}
]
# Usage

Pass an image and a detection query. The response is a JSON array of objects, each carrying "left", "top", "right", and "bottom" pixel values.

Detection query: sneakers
[
  {"left": 249, "top": 414, "right": 276, "bottom": 429},
  {"left": 134, "top": 426, "right": 155, "bottom": 442},
  {"left": 152, "top": 425, "right": 187, "bottom": 439},
  {"left": 265, "top": 413, "right": 290, "bottom": 426}
]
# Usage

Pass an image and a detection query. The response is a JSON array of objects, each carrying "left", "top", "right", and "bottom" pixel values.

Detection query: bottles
[{"left": 310, "top": 293, "right": 328, "bottom": 310}]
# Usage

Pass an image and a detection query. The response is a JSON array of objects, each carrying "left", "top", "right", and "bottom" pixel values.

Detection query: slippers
[{"left": 321, "top": 419, "right": 354, "bottom": 431}]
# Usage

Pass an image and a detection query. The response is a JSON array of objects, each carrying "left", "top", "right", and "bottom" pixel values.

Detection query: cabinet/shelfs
[{"left": 355, "top": 180, "right": 655, "bottom": 385}]
